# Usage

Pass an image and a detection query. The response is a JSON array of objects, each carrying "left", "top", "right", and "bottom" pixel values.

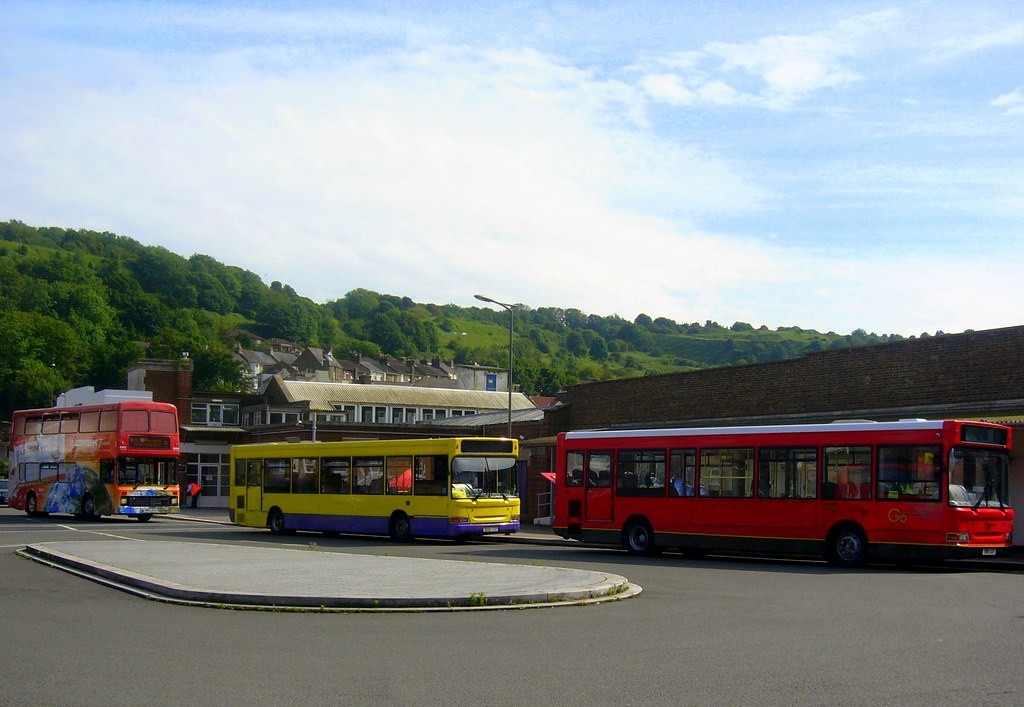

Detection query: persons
[{"left": 186, "top": 482, "right": 202, "bottom": 508}]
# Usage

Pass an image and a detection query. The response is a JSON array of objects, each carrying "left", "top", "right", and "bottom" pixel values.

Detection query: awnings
[
  {"left": 519, "top": 435, "right": 557, "bottom": 455},
  {"left": 179, "top": 424, "right": 246, "bottom": 446},
  {"left": 945, "top": 406, "right": 1021, "bottom": 439}
]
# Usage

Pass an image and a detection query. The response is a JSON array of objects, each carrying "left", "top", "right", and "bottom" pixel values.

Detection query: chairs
[
  {"left": 622, "top": 472, "right": 638, "bottom": 488},
  {"left": 573, "top": 470, "right": 583, "bottom": 483},
  {"left": 599, "top": 471, "right": 610, "bottom": 480},
  {"left": 709, "top": 490, "right": 720, "bottom": 496},
  {"left": 723, "top": 490, "right": 733, "bottom": 496}
]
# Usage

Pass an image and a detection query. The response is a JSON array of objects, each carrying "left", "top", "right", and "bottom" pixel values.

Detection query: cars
[{"left": 0, "top": 479, "right": 9, "bottom": 505}]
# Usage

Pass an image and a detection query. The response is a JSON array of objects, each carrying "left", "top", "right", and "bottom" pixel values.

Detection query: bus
[
  {"left": 538, "top": 417, "right": 1015, "bottom": 573},
  {"left": 228, "top": 436, "right": 521, "bottom": 544},
  {"left": 9, "top": 401, "right": 187, "bottom": 523}
]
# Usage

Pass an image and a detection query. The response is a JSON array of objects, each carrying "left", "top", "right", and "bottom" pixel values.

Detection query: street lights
[
  {"left": 60, "top": 393, "right": 67, "bottom": 407},
  {"left": 474, "top": 294, "right": 516, "bottom": 438}
]
